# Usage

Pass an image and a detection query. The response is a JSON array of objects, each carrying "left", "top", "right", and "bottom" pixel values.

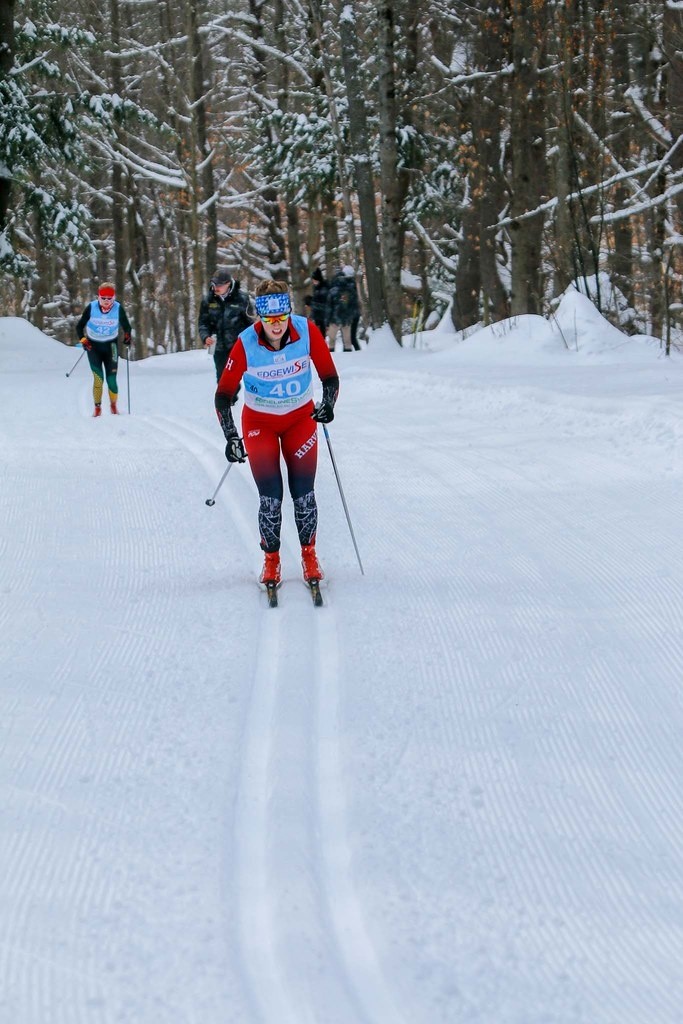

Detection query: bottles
[{"left": 208, "top": 334, "right": 217, "bottom": 355}]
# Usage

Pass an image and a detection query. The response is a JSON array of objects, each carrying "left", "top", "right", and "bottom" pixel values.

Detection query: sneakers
[
  {"left": 93, "top": 406, "right": 102, "bottom": 416},
  {"left": 110, "top": 405, "right": 118, "bottom": 414},
  {"left": 300, "top": 545, "right": 324, "bottom": 582},
  {"left": 259, "top": 550, "right": 281, "bottom": 584}
]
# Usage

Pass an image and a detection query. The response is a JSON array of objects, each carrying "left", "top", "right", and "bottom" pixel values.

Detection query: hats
[
  {"left": 209, "top": 269, "right": 231, "bottom": 284},
  {"left": 311, "top": 268, "right": 323, "bottom": 281},
  {"left": 342, "top": 266, "right": 355, "bottom": 278}
]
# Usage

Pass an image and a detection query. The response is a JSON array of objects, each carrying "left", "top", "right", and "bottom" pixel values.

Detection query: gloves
[
  {"left": 225, "top": 436, "right": 248, "bottom": 463},
  {"left": 80, "top": 337, "right": 92, "bottom": 351},
  {"left": 310, "top": 401, "right": 334, "bottom": 423},
  {"left": 124, "top": 334, "right": 131, "bottom": 344}
]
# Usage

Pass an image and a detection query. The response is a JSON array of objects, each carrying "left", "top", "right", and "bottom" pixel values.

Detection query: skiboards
[{"left": 257, "top": 575, "right": 328, "bottom": 608}]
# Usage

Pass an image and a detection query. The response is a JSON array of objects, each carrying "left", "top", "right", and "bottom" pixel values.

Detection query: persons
[
  {"left": 76, "top": 282, "right": 132, "bottom": 417},
  {"left": 303, "top": 265, "right": 364, "bottom": 353},
  {"left": 198, "top": 269, "right": 257, "bottom": 407},
  {"left": 214, "top": 278, "right": 339, "bottom": 608}
]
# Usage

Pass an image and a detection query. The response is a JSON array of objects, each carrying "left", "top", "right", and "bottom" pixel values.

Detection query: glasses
[
  {"left": 100, "top": 297, "right": 113, "bottom": 301},
  {"left": 259, "top": 313, "right": 290, "bottom": 325}
]
